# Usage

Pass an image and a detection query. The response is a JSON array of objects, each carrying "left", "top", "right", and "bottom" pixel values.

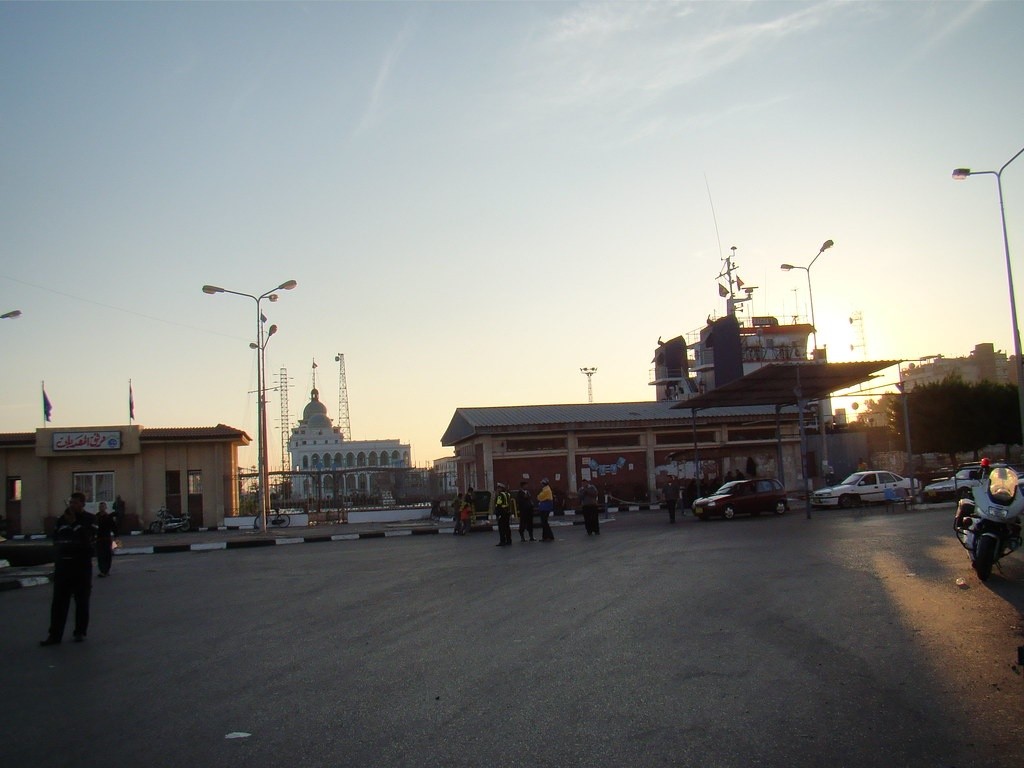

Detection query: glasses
[{"left": 70, "top": 501, "right": 78, "bottom": 504}]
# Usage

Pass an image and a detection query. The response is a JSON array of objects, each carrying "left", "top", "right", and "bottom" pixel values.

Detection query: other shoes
[
  {"left": 521, "top": 539, "right": 527, "bottom": 541},
  {"left": 587, "top": 531, "right": 592, "bottom": 535},
  {"left": 539, "top": 537, "right": 554, "bottom": 542},
  {"left": 72, "top": 635, "right": 83, "bottom": 642},
  {"left": 595, "top": 532, "right": 600, "bottom": 535},
  {"left": 97, "top": 572, "right": 110, "bottom": 577},
  {"left": 530, "top": 538, "right": 536, "bottom": 541},
  {"left": 40, "top": 635, "right": 61, "bottom": 645},
  {"left": 670, "top": 520, "right": 675, "bottom": 523},
  {"left": 496, "top": 542, "right": 511, "bottom": 546}
]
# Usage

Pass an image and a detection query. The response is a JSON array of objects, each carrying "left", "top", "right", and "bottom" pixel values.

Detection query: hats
[
  {"left": 497, "top": 483, "right": 505, "bottom": 487},
  {"left": 541, "top": 478, "right": 549, "bottom": 484}
]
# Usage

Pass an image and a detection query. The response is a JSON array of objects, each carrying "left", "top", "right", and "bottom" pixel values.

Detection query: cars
[
  {"left": 810, "top": 470, "right": 921, "bottom": 509},
  {"left": 923, "top": 464, "right": 1024, "bottom": 504},
  {"left": 692, "top": 478, "right": 788, "bottom": 521}
]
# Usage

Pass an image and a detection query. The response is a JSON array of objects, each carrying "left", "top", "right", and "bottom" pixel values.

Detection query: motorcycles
[
  {"left": 149, "top": 505, "right": 192, "bottom": 533},
  {"left": 952, "top": 458, "right": 1024, "bottom": 580}
]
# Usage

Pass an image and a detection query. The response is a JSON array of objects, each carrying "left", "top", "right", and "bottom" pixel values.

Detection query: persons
[
  {"left": 452, "top": 487, "right": 475, "bottom": 536},
  {"left": 537, "top": 478, "right": 554, "bottom": 541},
  {"left": 39, "top": 493, "right": 100, "bottom": 646},
  {"left": 493, "top": 483, "right": 513, "bottom": 546},
  {"left": 578, "top": 480, "right": 600, "bottom": 537},
  {"left": 724, "top": 469, "right": 746, "bottom": 483},
  {"left": 857, "top": 457, "right": 868, "bottom": 472},
  {"left": 112, "top": 495, "right": 126, "bottom": 537},
  {"left": 94, "top": 502, "right": 114, "bottom": 577},
  {"left": 517, "top": 481, "right": 537, "bottom": 543},
  {"left": 662, "top": 475, "right": 679, "bottom": 524}
]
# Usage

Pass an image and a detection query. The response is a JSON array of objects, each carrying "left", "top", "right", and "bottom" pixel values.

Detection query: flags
[
  {"left": 736, "top": 276, "right": 744, "bottom": 291},
  {"left": 44, "top": 390, "right": 52, "bottom": 422},
  {"left": 129, "top": 385, "right": 135, "bottom": 418},
  {"left": 719, "top": 283, "right": 729, "bottom": 297}
]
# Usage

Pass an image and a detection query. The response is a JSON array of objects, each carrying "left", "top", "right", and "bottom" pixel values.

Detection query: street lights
[
  {"left": 781, "top": 239, "right": 835, "bottom": 485},
  {"left": 249, "top": 324, "right": 277, "bottom": 511},
  {"left": 203, "top": 280, "right": 298, "bottom": 531},
  {"left": 580, "top": 367, "right": 598, "bottom": 403},
  {"left": 952, "top": 167, "right": 1024, "bottom": 415}
]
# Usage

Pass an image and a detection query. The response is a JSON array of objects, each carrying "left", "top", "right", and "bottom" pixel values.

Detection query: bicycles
[{"left": 254, "top": 509, "right": 291, "bottom": 529}]
